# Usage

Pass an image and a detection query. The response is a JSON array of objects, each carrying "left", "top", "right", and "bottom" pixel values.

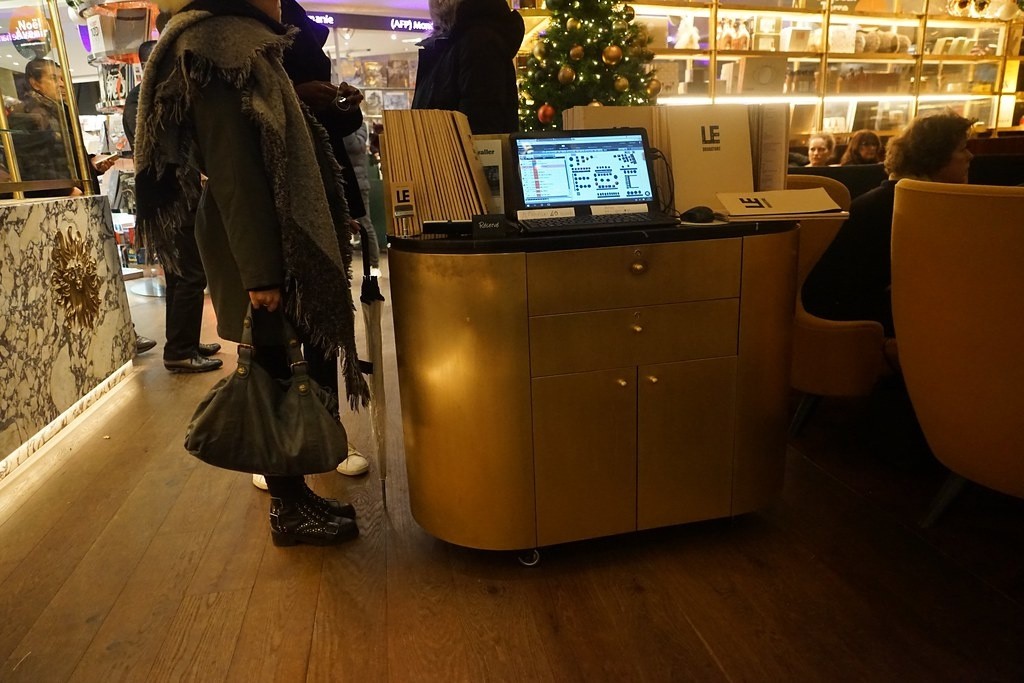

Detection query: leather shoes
[
  {"left": 135, "top": 335, "right": 156, "bottom": 353},
  {"left": 163, "top": 354, "right": 223, "bottom": 373},
  {"left": 196, "top": 343, "right": 220, "bottom": 356}
]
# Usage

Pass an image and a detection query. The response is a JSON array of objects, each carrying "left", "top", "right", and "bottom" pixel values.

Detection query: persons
[
  {"left": 804, "top": 133, "right": 835, "bottom": 167},
  {"left": 409, "top": 2, "right": 526, "bottom": 132},
  {"left": 122, "top": 39, "right": 223, "bottom": 373},
  {"left": 343, "top": 122, "right": 382, "bottom": 279},
  {"left": 801, "top": 114, "right": 972, "bottom": 329},
  {"left": 130, "top": 0, "right": 369, "bottom": 547},
  {"left": 369, "top": 124, "right": 383, "bottom": 156},
  {"left": 252, "top": 0, "right": 368, "bottom": 489},
  {"left": 841, "top": 130, "right": 879, "bottom": 165},
  {"left": 3, "top": 59, "right": 158, "bottom": 353}
]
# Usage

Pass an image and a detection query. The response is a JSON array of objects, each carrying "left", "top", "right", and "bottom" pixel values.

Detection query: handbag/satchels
[{"left": 183, "top": 299, "right": 347, "bottom": 476}]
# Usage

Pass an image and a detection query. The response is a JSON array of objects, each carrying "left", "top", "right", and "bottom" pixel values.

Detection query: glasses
[{"left": 860, "top": 141, "right": 878, "bottom": 146}]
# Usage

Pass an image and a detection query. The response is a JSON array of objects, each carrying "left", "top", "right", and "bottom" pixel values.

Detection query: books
[
  {"left": 381, "top": 108, "right": 505, "bottom": 235},
  {"left": 651, "top": 104, "right": 850, "bottom": 220}
]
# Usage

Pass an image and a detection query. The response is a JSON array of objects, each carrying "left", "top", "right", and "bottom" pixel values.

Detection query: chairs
[
  {"left": 785, "top": 174, "right": 887, "bottom": 437},
  {"left": 891, "top": 179, "right": 1024, "bottom": 530}
]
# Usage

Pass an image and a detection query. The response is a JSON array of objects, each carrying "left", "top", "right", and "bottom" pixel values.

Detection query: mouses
[{"left": 680, "top": 206, "right": 715, "bottom": 223}]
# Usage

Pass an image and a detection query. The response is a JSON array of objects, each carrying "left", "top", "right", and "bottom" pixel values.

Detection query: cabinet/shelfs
[
  {"left": 385, "top": 221, "right": 801, "bottom": 568},
  {"left": 512, "top": 0, "right": 1024, "bottom": 134}
]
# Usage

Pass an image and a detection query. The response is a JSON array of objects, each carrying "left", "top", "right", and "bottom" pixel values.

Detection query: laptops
[{"left": 509, "top": 127, "right": 682, "bottom": 236}]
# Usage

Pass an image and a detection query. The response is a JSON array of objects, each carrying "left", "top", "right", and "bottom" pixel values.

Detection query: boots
[{"left": 270, "top": 483, "right": 359, "bottom": 547}]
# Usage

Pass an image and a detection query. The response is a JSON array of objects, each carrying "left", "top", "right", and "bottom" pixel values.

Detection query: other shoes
[
  {"left": 337, "top": 443, "right": 369, "bottom": 476},
  {"left": 251, "top": 473, "right": 270, "bottom": 491}
]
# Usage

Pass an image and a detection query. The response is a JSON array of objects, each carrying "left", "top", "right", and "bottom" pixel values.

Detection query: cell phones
[{"left": 95, "top": 154, "right": 119, "bottom": 170}]
